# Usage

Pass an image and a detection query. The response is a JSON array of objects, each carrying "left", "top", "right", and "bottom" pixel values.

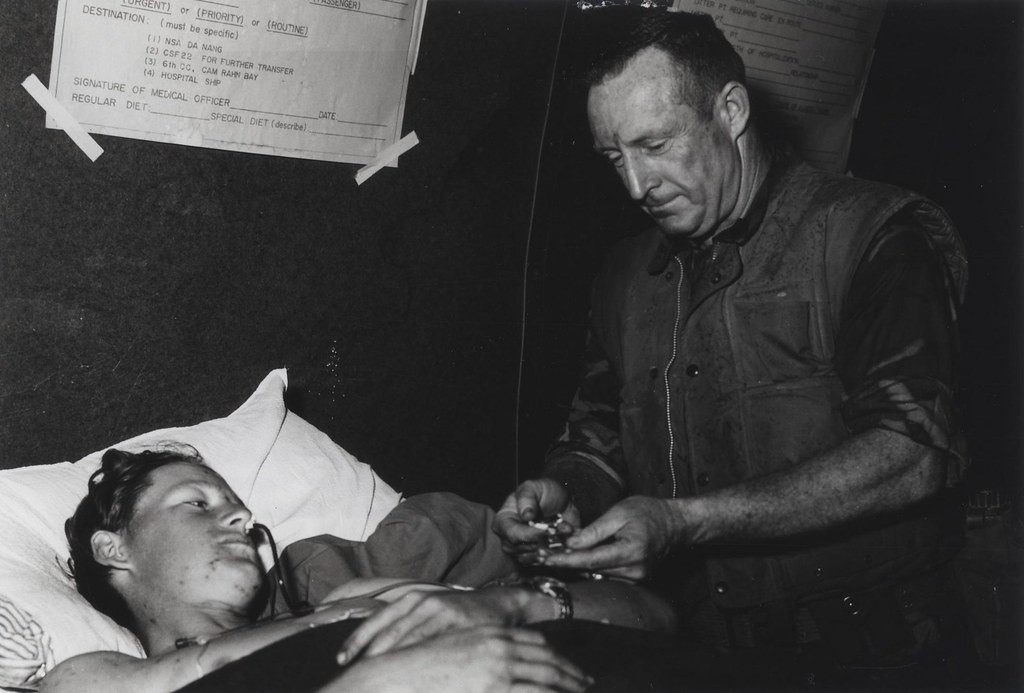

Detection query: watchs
[
  {"left": 524, "top": 573, "right": 573, "bottom": 618},
  {"left": 529, "top": 513, "right": 572, "bottom": 552}
]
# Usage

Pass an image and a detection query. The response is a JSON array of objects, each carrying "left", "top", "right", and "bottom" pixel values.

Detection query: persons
[
  {"left": 34, "top": 448, "right": 908, "bottom": 693},
  {"left": 491, "top": 10, "right": 985, "bottom": 693}
]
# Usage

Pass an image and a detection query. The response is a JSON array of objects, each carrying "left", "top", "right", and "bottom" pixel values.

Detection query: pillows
[{"left": 0, "top": 366, "right": 407, "bottom": 691}]
